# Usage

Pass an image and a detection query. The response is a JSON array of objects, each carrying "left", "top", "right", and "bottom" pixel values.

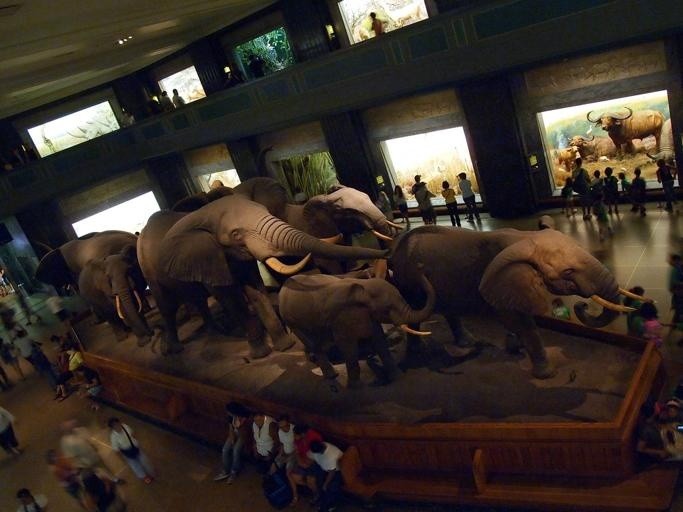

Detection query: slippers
[{"left": 52, "top": 394, "right": 69, "bottom": 403}]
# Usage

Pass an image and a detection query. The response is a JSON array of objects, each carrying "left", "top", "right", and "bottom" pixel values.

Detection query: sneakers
[
  {"left": 144, "top": 475, "right": 153, "bottom": 486},
  {"left": 289, "top": 495, "right": 340, "bottom": 511},
  {"left": 214, "top": 471, "right": 235, "bottom": 486}
]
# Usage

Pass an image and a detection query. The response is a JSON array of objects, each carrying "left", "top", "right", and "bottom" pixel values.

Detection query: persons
[
  {"left": 413, "top": 181, "right": 437, "bottom": 225},
  {"left": 160, "top": 90, "right": 175, "bottom": 112},
  {"left": 457, "top": 172, "right": 481, "bottom": 224},
  {"left": 171, "top": 88, "right": 185, "bottom": 108},
  {"left": 223, "top": 63, "right": 247, "bottom": 89},
  {"left": 0, "top": 267, "right": 105, "bottom": 413},
  {"left": 124, "top": 110, "right": 135, "bottom": 124},
  {"left": 245, "top": 54, "right": 265, "bottom": 78},
  {"left": 556, "top": 157, "right": 677, "bottom": 243},
  {"left": 369, "top": 12, "right": 385, "bottom": 38},
  {"left": 46, "top": 415, "right": 153, "bottom": 511},
  {"left": 408, "top": 173, "right": 422, "bottom": 195},
  {"left": 377, "top": 191, "right": 398, "bottom": 238},
  {"left": 393, "top": 184, "right": 411, "bottom": 229},
  {"left": 0, "top": 408, "right": 23, "bottom": 459},
  {"left": 145, "top": 95, "right": 161, "bottom": 118},
  {"left": 623, "top": 251, "right": 683, "bottom": 466},
  {"left": 551, "top": 297, "right": 571, "bottom": 321},
  {"left": 293, "top": 187, "right": 308, "bottom": 202},
  {"left": 213, "top": 402, "right": 347, "bottom": 512},
  {"left": 439, "top": 180, "right": 461, "bottom": 227},
  {"left": 14, "top": 489, "right": 49, "bottom": 512}
]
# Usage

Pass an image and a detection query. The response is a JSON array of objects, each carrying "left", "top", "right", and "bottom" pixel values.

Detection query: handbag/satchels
[{"left": 119, "top": 445, "right": 141, "bottom": 460}]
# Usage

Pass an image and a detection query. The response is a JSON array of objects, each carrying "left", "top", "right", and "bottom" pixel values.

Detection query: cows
[{"left": 585, "top": 108, "right": 664, "bottom": 158}]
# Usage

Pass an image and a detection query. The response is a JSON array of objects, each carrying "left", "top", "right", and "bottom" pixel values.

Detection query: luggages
[{"left": 261, "top": 471, "right": 294, "bottom": 511}]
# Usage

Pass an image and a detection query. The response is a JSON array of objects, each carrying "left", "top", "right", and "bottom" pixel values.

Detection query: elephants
[
  {"left": 390, "top": 226, "right": 653, "bottom": 380},
  {"left": 31, "top": 231, "right": 155, "bottom": 315},
  {"left": 136, "top": 175, "right": 436, "bottom": 391},
  {"left": 76, "top": 244, "right": 154, "bottom": 341}
]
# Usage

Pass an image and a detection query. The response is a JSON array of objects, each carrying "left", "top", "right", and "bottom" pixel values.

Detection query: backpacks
[
  {"left": 604, "top": 176, "right": 618, "bottom": 203},
  {"left": 572, "top": 169, "right": 586, "bottom": 195}
]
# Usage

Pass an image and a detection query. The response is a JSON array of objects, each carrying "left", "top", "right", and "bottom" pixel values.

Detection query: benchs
[{"left": 80, "top": 358, "right": 683, "bottom": 512}]
